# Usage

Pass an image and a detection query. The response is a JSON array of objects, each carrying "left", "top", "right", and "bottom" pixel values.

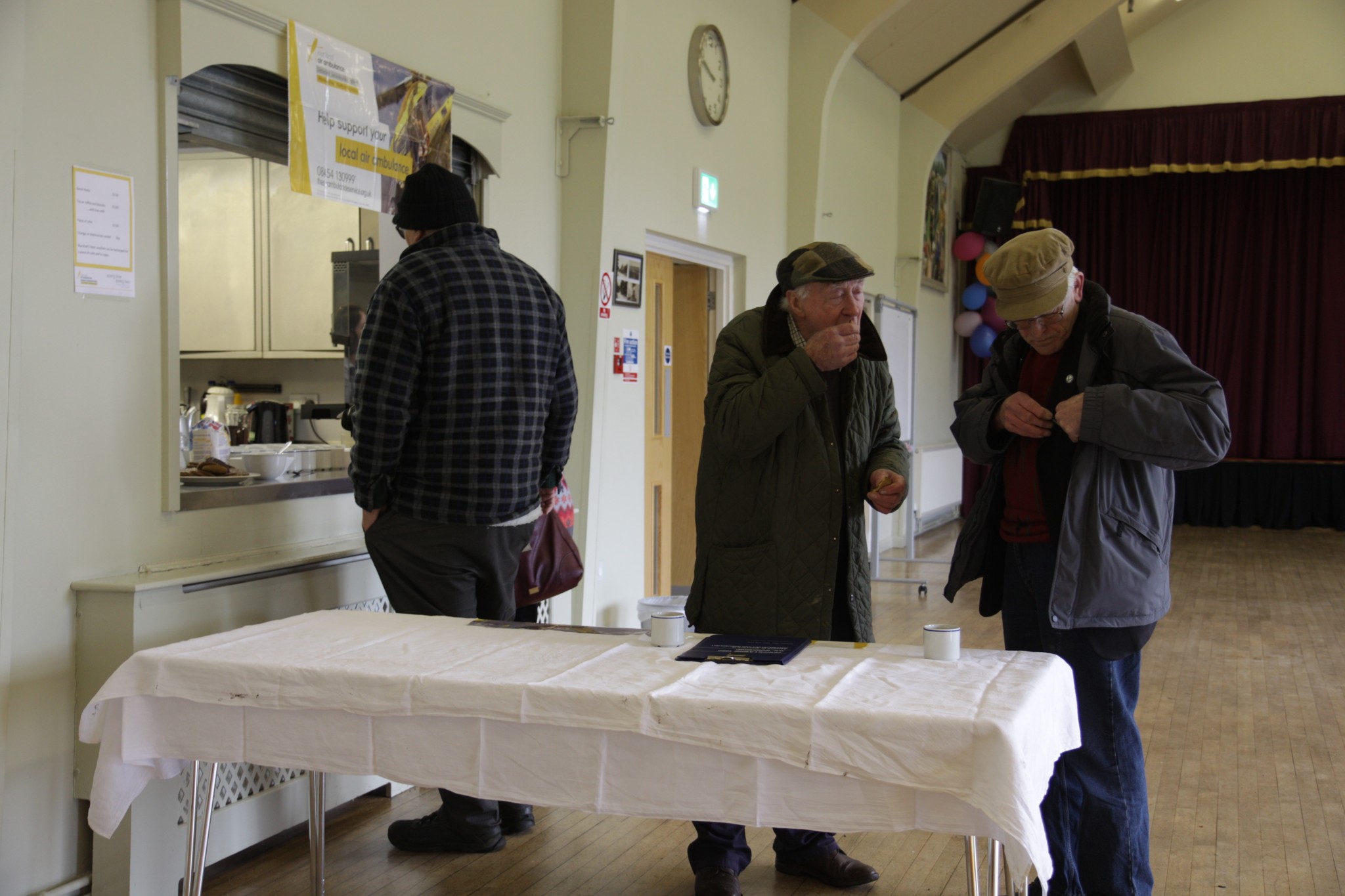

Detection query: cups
[
  {"left": 229, "top": 443, "right": 350, "bottom": 473},
  {"left": 923, "top": 624, "right": 961, "bottom": 662},
  {"left": 651, "top": 612, "right": 685, "bottom": 647}
]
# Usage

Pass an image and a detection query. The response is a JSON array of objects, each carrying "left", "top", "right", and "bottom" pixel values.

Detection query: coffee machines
[
  {"left": 246, "top": 400, "right": 290, "bottom": 444},
  {"left": 202, "top": 386, "right": 234, "bottom": 427}
]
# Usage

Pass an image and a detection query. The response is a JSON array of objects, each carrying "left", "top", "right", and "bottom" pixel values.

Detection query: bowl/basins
[{"left": 231, "top": 453, "right": 295, "bottom": 480}]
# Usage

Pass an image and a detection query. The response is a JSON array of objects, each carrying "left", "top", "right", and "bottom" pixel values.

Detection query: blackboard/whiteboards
[{"left": 875, "top": 294, "right": 917, "bottom": 444}]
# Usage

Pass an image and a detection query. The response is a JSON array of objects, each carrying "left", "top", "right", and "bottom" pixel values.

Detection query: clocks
[{"left": 684, "top": 20, "right": 734, "bottom": 130}]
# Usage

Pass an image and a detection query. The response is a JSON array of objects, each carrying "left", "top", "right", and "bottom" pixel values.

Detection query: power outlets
[{"left": 300, "top": 393, "right": 319, "bottom": 405}]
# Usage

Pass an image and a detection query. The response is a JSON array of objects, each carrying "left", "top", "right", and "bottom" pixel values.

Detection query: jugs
[{"left": 180, "top": 403, "right": 198, "bottom": 450}]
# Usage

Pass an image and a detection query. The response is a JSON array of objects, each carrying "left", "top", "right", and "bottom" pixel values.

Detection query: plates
[{"left": 180, "top": 473, "right": 261, "bottom": 485}]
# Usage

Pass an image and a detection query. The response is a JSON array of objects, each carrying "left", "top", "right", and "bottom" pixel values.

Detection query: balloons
[{"left": 953, "top": 232, "right": 1007, "bottom": 359}]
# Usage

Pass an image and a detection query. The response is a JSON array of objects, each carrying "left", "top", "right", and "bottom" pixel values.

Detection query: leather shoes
[
  {"left": 774, "top": 848, "right": 879, "bottom": 888},
  {"left": 694, "top": 865, "right": 741, "bottom": 896}
]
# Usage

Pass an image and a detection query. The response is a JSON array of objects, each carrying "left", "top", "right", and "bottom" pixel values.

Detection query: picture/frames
[
  {"left": 920, "top": 141, "right": 956, "bottom": 294},
  {"left": 611, "top": 246, "right": 645, "bottom": 310}
]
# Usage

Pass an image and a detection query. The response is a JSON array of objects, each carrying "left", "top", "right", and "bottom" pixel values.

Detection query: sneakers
[
  {"left": 501, "top": 812, "right": 536, "bottom": 836},
  {"left": 388, "top": 812, "right": 506, "bottom": 854}
]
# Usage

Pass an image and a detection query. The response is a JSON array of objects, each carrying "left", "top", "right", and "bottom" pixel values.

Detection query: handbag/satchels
[{"left": 513, "top": 506, "right": 584, "bottom": 608}]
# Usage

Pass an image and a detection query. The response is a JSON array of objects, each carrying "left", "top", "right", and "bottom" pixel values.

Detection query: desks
[{"left": 76, "top": 605, "right": 1085, "bottom": 896}]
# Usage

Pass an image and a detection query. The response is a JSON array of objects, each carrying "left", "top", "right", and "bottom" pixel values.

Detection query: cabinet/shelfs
[
  {"left": 256, "top": 157, "right": 366, "bottom": 364},
  {"left": 178, "top": 148, "right": 258, "bottom": 359}
]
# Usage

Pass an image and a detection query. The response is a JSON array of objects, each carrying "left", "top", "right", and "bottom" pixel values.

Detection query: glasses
[
  {"left": 394, "top": 224, "right": 405, "bottom": 240},
  {"left": 1007, "top": 299, "right": 1065, "bottom": 331}
]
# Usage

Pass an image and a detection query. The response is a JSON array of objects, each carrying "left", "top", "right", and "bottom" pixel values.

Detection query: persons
[
  {"left": 942, "top": 228, "right": 1227, "bottom": 896},
  {"left": 687, "top": 243, "right": 912, "bottom": 896},
  {"left": 346, "top": 161, "right": 579, "bottom": 850}
]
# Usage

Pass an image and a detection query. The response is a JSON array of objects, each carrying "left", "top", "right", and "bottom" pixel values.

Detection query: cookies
[{"left": 181, "top": 456, "right": 247, "bottom": 476}]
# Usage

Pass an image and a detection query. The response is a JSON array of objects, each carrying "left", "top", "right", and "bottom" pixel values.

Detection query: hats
[
  {"left": 776, "top": 242, "right": 875, "bottom": 290},
  {"left": 391, "top": 163, "right": 479, "bottom": 231},
  {"left": 983, "top": 228, "right": 1074, "bottom": 320}
]
